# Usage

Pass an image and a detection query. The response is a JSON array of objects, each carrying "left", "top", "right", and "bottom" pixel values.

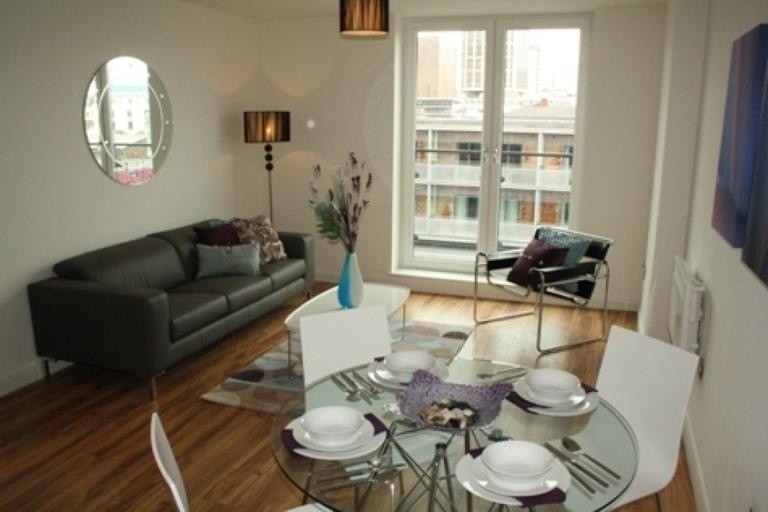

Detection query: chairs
[
  {"left": 509, "top": 324, "right": 700, "bottom": 512},
  {"left": 472, "top": 226, "right": 615, "bottom": 354},
  {"left": 151, "top": 411, "right": 338, "bottom": 512},
  {"left": 300, "top": 306, "right": 465, "bottom": 511}
]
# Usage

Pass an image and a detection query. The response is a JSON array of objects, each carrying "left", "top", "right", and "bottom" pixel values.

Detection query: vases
[{"left": 337, "top": 252, "right": 364, "bottom": 309}]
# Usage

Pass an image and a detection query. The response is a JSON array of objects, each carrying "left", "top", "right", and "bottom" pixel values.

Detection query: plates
[
  {"left": 455, "top": 452, "right": 572, "bottom": 506},
  {"left": 283, "top": 416, "right": 387, "bottom": 460},
  {"left": 514, "top": 376, "right": 586, "bottom": 407},
  {"left": 368, "top": 359, "right": 448, "bottom": 389},
  {"left": 470, "top": 452, "right": 561, "bottom": 497},
  {"left": 292, "top": 416, "right": 375, "bottom": 451},
  {"left": 375, "top": 363, "right": 410, "bottom": 383},
  {"left": 506, "top": 390, "right": 599, "bottom": 417},
  {"left": 393, "top": 369, "right": 515, "bottom": 433}
]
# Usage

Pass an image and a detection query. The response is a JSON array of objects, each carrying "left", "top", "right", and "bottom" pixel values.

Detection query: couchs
[{"left": 26, "top": 218, "right": 314, "bottom": 400}]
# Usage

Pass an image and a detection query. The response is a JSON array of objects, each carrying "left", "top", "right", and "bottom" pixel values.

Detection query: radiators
[{"left": 668, "top": 254, "right": 705, "bottom": 354}]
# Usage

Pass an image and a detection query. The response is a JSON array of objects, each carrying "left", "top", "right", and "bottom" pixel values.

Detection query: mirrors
[{"left": 83, "top": 55, "right": 173, "bottom": 187}]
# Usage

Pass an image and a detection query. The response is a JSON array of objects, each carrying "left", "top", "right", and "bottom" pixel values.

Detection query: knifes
[
  {"left": 340, "top": 371, "right": 372, "bottom": 405},
  {"left": 316, "top": 463, "right": 404, "bottom": 483},
  {"left": 545, "top": 442, "right": 610, "bottom": 488}
]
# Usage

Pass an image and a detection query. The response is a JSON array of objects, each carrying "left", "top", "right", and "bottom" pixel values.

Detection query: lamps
[
  {"left": 243, "top": 110, "right": 291, "bottom": 227},
  {"left": 338, "top": 0, "right": 390, "bottom": 37}
]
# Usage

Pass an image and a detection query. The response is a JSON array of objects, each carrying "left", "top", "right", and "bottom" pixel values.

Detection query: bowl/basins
[
  {"left": 482, "top": 440, "right": 554, "bottom": 491},
  {"left": 385, "top": 350, "right": 435, "bottom": 378},
  {"left": 525, "top": 367, "right": 580, "bottom": 399},
  {"left": 301, "top": 405, "right": 364, "bottom": 440}
]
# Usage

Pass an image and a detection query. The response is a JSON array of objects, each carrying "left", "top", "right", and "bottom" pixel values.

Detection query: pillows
[
  {"left": 196, "top": 241, "right": 263, "bottom": 277},
  {"left": 190, "top": 223, "right": 241, "bottom": 246},
  {"left": 507, "top": 238, "right": 571, "bottom": 294},
  {"left": 231, "top": 214, "right": 287, "bottom": 266},
  {"left": 538, "top": 228, "right": 593, "bottom": 292}
]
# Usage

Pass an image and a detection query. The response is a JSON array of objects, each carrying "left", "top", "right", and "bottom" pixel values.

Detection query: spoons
[
  {"left": 561, "top": 436, "right": 621, "bottom": 479},
  {"left": 320, "top": 465, "right": 402, "bottom": 494},
  {"left": 476, "top": 368, "right": 524, "bottom": 380},
  {"left": 330, "top": 374, "right": 360, "bottom": 401}
]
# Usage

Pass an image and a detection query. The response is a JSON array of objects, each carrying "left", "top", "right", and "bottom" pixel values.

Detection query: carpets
[{"left": 200, "top": 318, "right": 475, "bottom": 416}]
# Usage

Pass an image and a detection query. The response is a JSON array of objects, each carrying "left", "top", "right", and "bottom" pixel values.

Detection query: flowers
[{"left": 307, "top": 150, "right": 373, "bottom": 253}]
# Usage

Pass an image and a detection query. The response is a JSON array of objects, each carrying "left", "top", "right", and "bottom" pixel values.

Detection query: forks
[
  {"left": 310, "top": 457, "right": 389, "bottom": 473},
  {"left": 352, "top": 369, "right": 382, "bottom": 394}
]
